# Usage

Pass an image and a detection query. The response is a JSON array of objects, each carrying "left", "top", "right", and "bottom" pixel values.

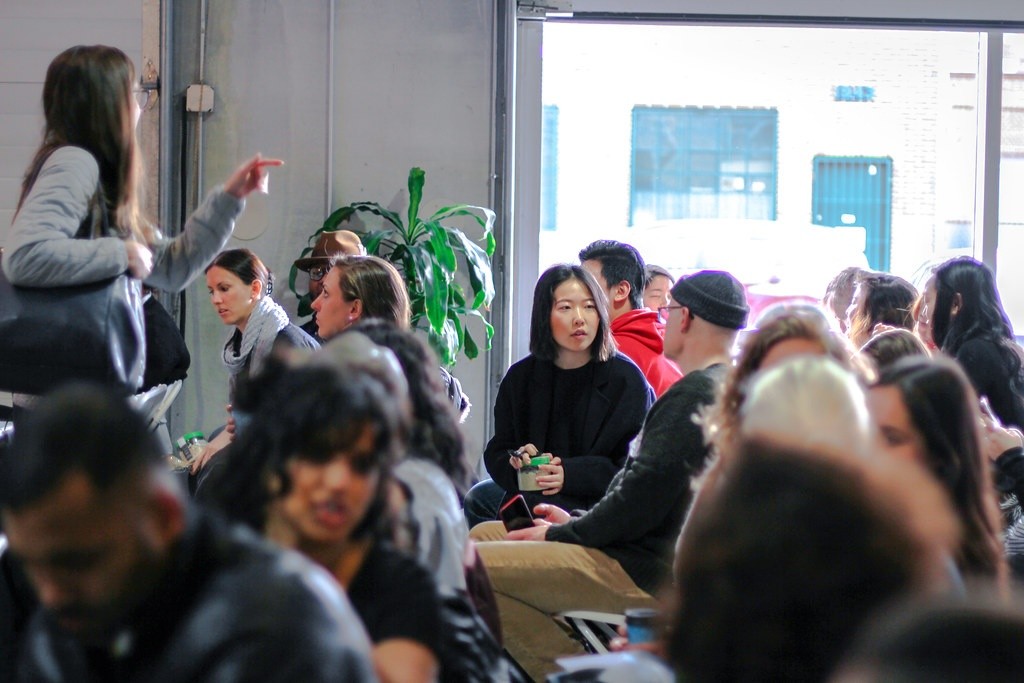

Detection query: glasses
[
  {"left": 308, "top": 267, "right": 330, "bottom": 281},
  {"left": 658, "top": 306, "right": 694, "bottom": 324}
]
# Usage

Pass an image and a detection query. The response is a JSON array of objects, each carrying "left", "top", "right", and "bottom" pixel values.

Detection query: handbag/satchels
[{"left": 0, "top": 146, "right": 136, "bottom": 395}]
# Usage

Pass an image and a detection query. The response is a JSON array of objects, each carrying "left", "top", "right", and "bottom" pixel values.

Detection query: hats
[
  {"left": 294, "top": 229, "right": 364, "bottom": 272},
  {"left": 670, "top": 269, "right": 750, "bottom": 328}
]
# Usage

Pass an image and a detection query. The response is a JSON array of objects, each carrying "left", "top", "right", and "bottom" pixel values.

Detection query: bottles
[
  {"left": 517, "top": 456, "right": 550, "bottom": 490},
  {"left": 176, "top": 432, "right": 208, "bottom": 462}
]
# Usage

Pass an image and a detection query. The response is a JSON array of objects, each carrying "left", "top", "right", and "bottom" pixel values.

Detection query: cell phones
[{"left": 500, "top": 495, "right": 535, "bottom": 532}]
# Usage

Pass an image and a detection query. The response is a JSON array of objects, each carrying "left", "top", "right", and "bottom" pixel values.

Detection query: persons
[
  {"left": 465, "top": 240, "right": 1024, "bottom": 683},
  {"left": 0, "top": 230, "right": 503, "bottom": 683},
  {"left": 0, "top": 45, "right": 284, "bottom": 460}
]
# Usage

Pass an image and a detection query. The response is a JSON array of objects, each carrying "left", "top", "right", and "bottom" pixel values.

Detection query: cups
[{"left": 626, "top": 609, "right": 661, "bottom": 644}]
